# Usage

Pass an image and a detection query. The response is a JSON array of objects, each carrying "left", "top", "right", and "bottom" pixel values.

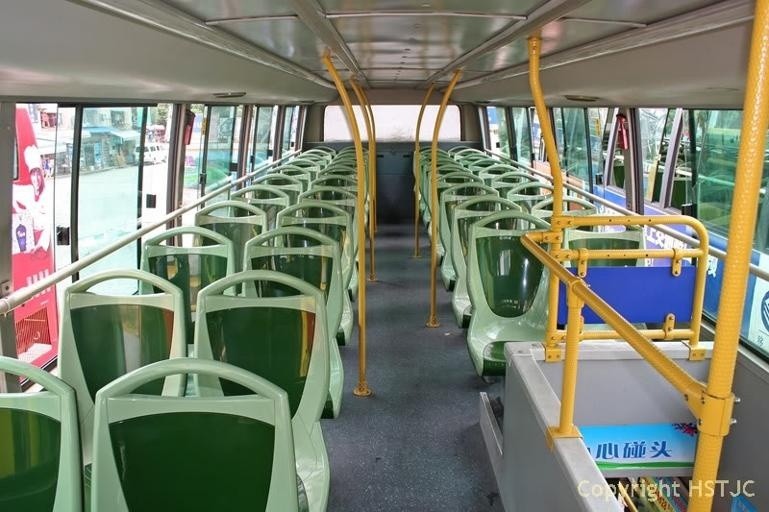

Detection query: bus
[
  {"left": 486, "top": 106, "right": 769, "bottom": 366},
  {"left": 177, "top": 102, "right": 303, "bottom": 190},
  {"left": 12, "top": 106, "right": 60, "bottom": 391}
]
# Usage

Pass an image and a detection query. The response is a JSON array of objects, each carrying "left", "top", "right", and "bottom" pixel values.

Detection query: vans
[{"left": 134, "top": 144, "right": 168, "bottom": 166}]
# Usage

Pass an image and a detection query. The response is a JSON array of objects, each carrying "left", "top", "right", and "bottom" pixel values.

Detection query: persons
[{"left": 12, "top": 167, "right": 54, "bottom": 256}]
